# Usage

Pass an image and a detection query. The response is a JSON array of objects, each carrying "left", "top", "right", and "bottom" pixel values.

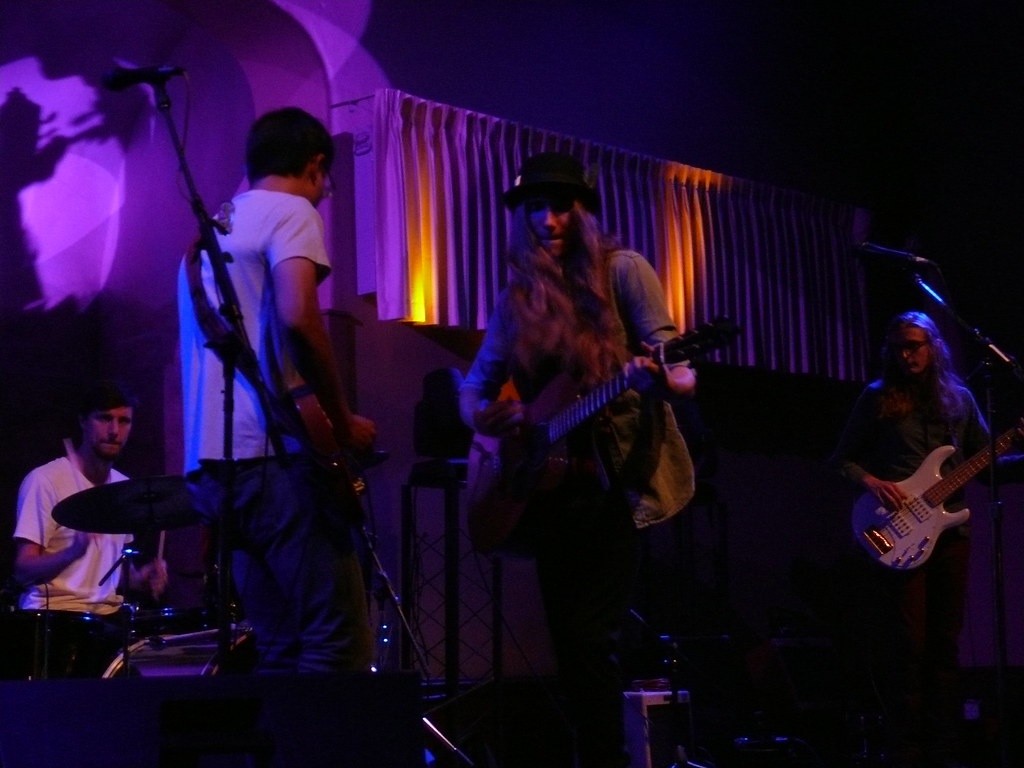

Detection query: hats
[{"left": 501, "top": 151, "right": 601, "bottom": 217}]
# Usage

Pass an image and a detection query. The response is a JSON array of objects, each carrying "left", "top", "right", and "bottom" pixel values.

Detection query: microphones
[
  {"left": 100, "top": 64, "right": 185, "bottom": 93},
  {"left": 858, "top": 243, "right": 927, "bottom": 264}
]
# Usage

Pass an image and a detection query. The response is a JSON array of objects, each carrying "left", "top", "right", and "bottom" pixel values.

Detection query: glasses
[
  {"left": 321, "top": 161, "right": 336, "bottom": 199},
  {"left": 524, "top": 193, "right": 574, "bottom": 212},
  {"left": 888, "top": 340, "right": 928, "bottom": 355}
]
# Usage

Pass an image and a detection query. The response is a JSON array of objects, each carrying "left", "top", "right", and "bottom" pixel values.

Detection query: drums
[{"left": 101, "top": 620, "right": 255, "bottom": 679}]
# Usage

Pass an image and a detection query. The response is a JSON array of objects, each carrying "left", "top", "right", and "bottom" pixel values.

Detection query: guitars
[
  {"left": 455, "top": 311, "right": 741, "bottom": 562},
  {"left": 847, "top": 375, "right": 1024, "bottom": 574}
]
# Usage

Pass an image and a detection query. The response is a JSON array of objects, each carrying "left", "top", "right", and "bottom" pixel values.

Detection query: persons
[
  {"left": 824, "top": 311, "right": 1007, "bottom": 657},
  {"left": 459, "top": 152, "right": 702, "bottom": 670},
  {"left": 177, "top": 107, "right": 378, "bottom": 674},
  {"left": 13, "top": 381, "right": 170, "bottom": 680}
]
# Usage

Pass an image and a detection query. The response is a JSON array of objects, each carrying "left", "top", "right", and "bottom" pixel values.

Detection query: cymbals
[{"left": 50, "top": 471, "right": 213, "bottom": 537}]
